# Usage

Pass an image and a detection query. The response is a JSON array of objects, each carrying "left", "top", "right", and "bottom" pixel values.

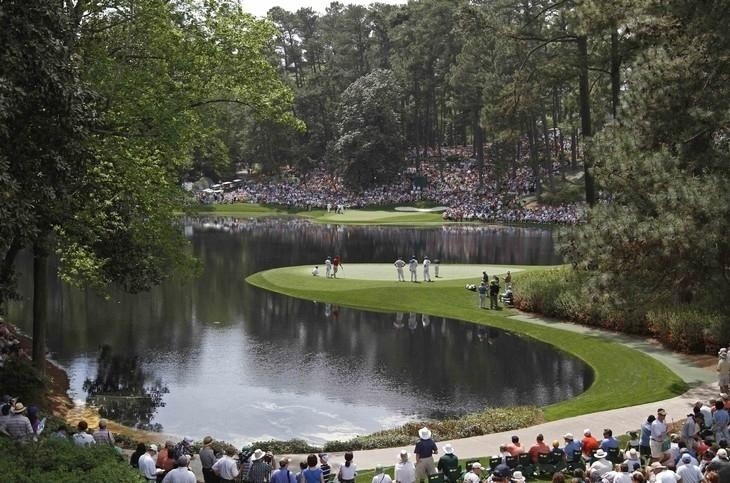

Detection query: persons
[
  {"left": 465, "top": 270, "right": 514, "bottom": 309},
  {"left": 409, "top": 254, "right": 418, "bottom": 282},
  {"left": 716, "top": 346, "right": 730, "bottom": 392},
  {"left": 423, "top": 255, "right": 433, "bottom": 282},
  {"left": 392, "top": 311, "right": 499, "bottom": 346},
  {"left": 311, "top": 265, "right": 320, "bottom": 276},
  {"left": 434, "top": 255, "right": 440, "bottom": 277},
  {"left": 323, "top": 255, "right": 331, "bottom": 278},
  {"left": 331, "top": 254, "right": 343, "bottom": 279},
  {"left": 394, "top": 255, "right": 406, "bottom": 281}
]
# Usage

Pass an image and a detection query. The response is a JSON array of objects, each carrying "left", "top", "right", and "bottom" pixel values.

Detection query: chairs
[{"left": 427, "top": 441, "right": 653, "bottom": 483}]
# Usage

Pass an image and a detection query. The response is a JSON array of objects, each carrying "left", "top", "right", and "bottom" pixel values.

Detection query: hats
[
  {"left": 717, "top": 448, "right": 728, "bottom": 459},
  {"left": 417, "top": 427, "right": 433, "bottom": 442},
  {"left": 651, "top": 461, "right": 667, "bottom": 470},
  {"left": 720, "top": 392, "right": 729, "bottom": 399},
  {"left": 397, "top": 450, "right": 412, "bottom": 462},
  {"left": 10, "top": 402, "right": 27, "bottom": 415},
  {"left": 241, "top": 445, "right": 266, "bottom": 461},
  {"left": 148, "top": 444, "right": 158, "bottom": 454},
  {"left": 657, "top": 409, "right": 668, "bottom": 416},
  {"left": 279, "top": 457, "right": 292, "bottom": 466},
  {"left": 203, "top": 436, "right": 215, "bottom": 448},
  {"left": 625, "top": 448, "right": 641, "bottom": 460},
  {"left": 375, "top": 464, "right": 385, "bottom": 474},
  {"left": 679, "top": 447, "right": 691, "bottom": 463},
  {"left": 717, "top": 347, "right": 728, "bottom": 360},
  {"left": 562, "top": 432, "right": 574, "bottom": 440},
  {"left": 442, "top": 444, "right": 455, "bottom": 455},
  {"left": 318, "top": 453, "right": 328, "bottom": 462},
  {"left": 584, "top": 428, "right": 592, "bottom": 436},
  {"left": 183, "top": 435, "right": 194, "bottom": 447},
  {"left": 593, "top": 448, "right": 608, "bottom": 459},
  {"left": 471, "top": 463, "right": 526, "bottom": 482}
]
